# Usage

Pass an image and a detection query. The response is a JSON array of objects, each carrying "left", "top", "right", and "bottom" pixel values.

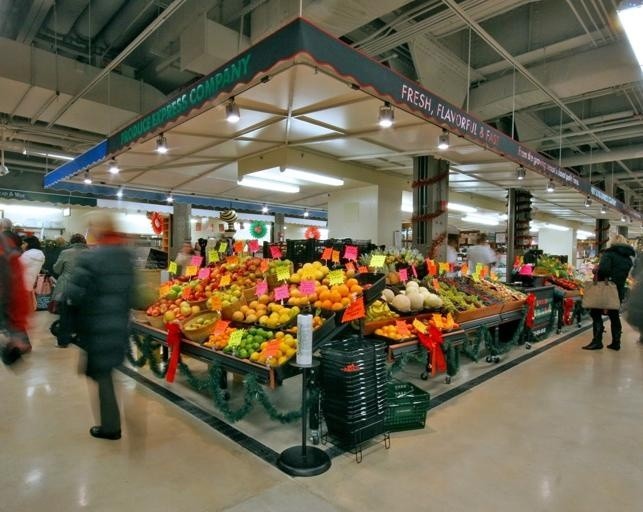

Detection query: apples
[
  {"left": 146, "top": 298, "right": 200, "bottom": 323},
  {"left": 167, "top": 279, "right": 198, "bottom": 298}
]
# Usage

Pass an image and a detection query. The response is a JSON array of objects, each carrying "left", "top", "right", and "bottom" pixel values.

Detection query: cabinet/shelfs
[
  {"left": 544, "top": 269, "right": 633, "bottom": 334},
  {"left": 401, "top": 223, "right": 413, "bottom": 249},
  {"left": 364, "top": 278, "right": 527, "bottom": 384},
  {"left": 129, "top": 268, "right": 385, "bottom": 445},
  {"left": 159, "top": 213, "right": 173, "bottom": 268}
]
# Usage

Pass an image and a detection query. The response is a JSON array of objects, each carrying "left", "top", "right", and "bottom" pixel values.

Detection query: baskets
[
  {"left": 130, "top": 308, "right": 148, "bottom": 324},
  {"left": 180, "top": 310, "right": 221, "bottom": 343},
  {"left": 188, "top": 299, "right": 210, "bottom": 310},
  {"left": 221, "top": 298, "right": 243, "bottom": 319},
  {"left": 385, "top": 381, "right": 430, "bottom": 400},
  {"left": 386, "top": 401, "right": 431, "bottom": 434},
  {"left": 286, "top": 239, "right": 373, "bottom": 261},
  {"left": 234, "top": 283, "right": 259, "bottom": 301},
  {"left": 266, "top": 267, "right": 289, "bottom": 289},
  {"left": 317, "top": 337, "right": 391, "bottom": 446},
  {"left": 147, "top": 315, "right": 164, "bottom": 330}
]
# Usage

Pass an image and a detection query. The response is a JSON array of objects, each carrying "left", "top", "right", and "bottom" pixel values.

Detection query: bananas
[{"left": 365, "top": 299, "right": 397, "bottom": 322}]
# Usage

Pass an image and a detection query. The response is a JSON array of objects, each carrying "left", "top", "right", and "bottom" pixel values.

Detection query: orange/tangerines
[{"left": 222, "top": 327, "right": 272, "bottom": 359}]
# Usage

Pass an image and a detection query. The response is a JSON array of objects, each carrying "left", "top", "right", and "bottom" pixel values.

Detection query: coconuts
[{"left": 382, "top": 280, "right": 441, "bottom": 311}]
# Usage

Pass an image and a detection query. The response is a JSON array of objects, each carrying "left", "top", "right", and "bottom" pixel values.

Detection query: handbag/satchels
[
  {"left": 581, "top": 280, "right": 621, "bottom": 311},
  {"left": 49, "top": 308, "right": 93, "bottom": 348},
  {"left": 35, "top": 274, "right": 53, "bottom": 296}
]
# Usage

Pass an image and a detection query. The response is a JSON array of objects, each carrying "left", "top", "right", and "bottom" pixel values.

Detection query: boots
[
  {"left": 582, "top": 320, "right": 603, "bottom": 350},
  {"left": 607, "top": 319, "right": 621, "bottom": 351}
]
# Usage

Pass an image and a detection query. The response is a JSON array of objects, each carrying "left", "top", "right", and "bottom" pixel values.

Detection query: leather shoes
[{"left": 88, "top": 424, "right": 122, "bottom": 440}]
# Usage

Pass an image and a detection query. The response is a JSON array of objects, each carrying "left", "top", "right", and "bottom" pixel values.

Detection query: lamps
[
  {"left": 547, "top": 178, "right": 627, "bottom": 223},
  {"left": 63, "top": 197, "right": 71, "bottom": 217},
  {"left": 437, "top": 128, "right": 451, "bottom": 150},
  {"left": 156, "top": 133, "right": 168, "bottom": 152},
  {"left": 84, "top": 169, "right": 92, "bottom": 184},
  {"left": 226, "top": 96, "right": 240, "bottom": 124},
  {"left": 378, "top": 101, "right": 395, "bottom": 128},
  {"left": 516, "top": 164, "right": 525, "bottom": 181},
  {"left": 109, "top": 157, "right": 120, "bottom": 173}
]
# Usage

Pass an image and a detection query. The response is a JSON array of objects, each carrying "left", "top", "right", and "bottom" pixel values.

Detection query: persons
[
  {"left": 56, "top": 211, "right": 134, "bottom": 440},
  {"left": 177, "top": 243, "right": 193, "bottom": 277},
  {"left": 446, "top": 239, "right": 460, "bottom": 277},
  {"left": 465, "top": 234, "right": 496, "bottom": 277},
  {"left": 1, "top": 218, "right": 46, "bottom": 357},
  {"left": 48, "top": 237, "right": 66, "bottom": 278},
  {"left": 582, "top": 234, "right": 634, "bottom": 350},
  {"left": 628, "top": 253, "right": 642, "bottom": 346},
  {"left": 53, "top": 234, "right": 88, "bottom": 347}
]
